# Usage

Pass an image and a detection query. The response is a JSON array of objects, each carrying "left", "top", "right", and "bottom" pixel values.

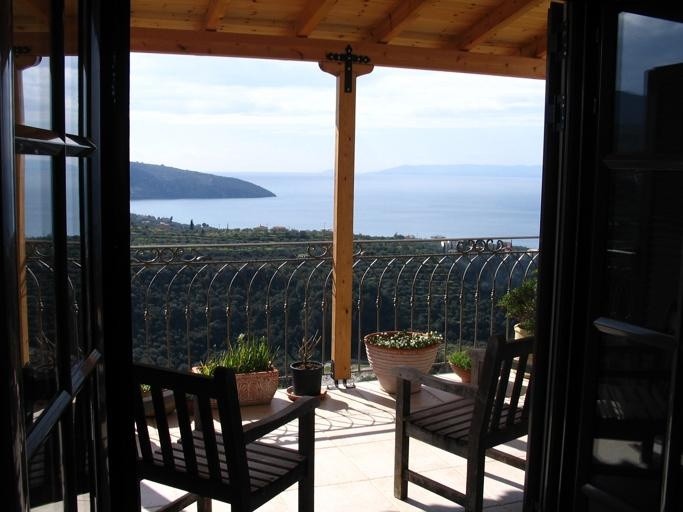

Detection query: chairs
[
  {"left": 390, "top": 331, "right": 547, "bottom": 512},
  {"left": 128, "top": 361, "right": 314, "bottom": 511}
]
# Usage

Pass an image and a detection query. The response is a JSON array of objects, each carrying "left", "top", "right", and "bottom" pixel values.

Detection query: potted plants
[
  {"left": 289, "top": 328, "right": 325, "bottom": 391},
  {"left": 500, "top": 271, "right": 539, "bottom": 368},
  {"left": 132, "top": 337, "right": 277, "bottom": 411},
  {"left": 364, "top": 329, "right": 442, "bottom": 395},
  {"left": 444, "top": 345, "right": 473, "bottom": 383}
]
